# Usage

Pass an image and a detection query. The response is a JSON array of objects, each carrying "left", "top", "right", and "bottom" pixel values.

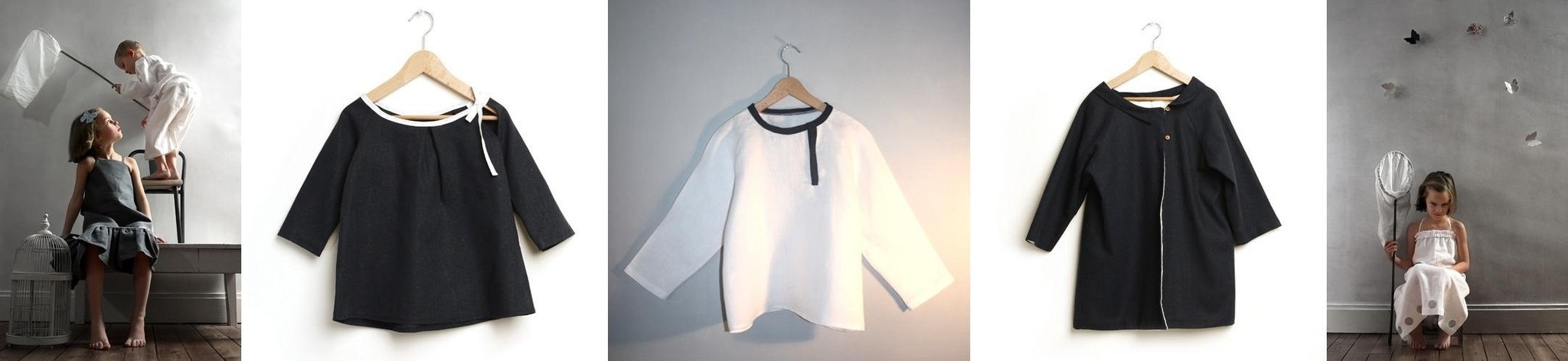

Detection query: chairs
[
  {"left": 124, "top": 144, "right": 191, "bottom": 248},
  {"left": 1400, "top": 306, "right": 1468, "bottom": 347}
]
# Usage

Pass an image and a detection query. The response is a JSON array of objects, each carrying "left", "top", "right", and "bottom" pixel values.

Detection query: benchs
[{"left": 66, "top": 229, "right": 240, "bottom": 331}]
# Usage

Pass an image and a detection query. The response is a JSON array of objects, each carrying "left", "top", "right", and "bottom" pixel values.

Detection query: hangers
[
  {"left": 358, "top": 6, "right": 503, "bottom": 128},
  {"left": 1096, "top": 13, "right": 1200, "bottom": 109},
  {"left": 752, "top": 39, "right": 835, "bottom": 123}
]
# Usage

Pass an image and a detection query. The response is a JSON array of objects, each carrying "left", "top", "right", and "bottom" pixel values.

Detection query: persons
[
  {"left": 1384, "top": 171, "right": 1470, "bottom": 350},
  {"left": 49, "top": 108, "right": 167, "bottom": 351},
  {"left": 111, "top": 41, "right": 192, "bottom": 179}
]
